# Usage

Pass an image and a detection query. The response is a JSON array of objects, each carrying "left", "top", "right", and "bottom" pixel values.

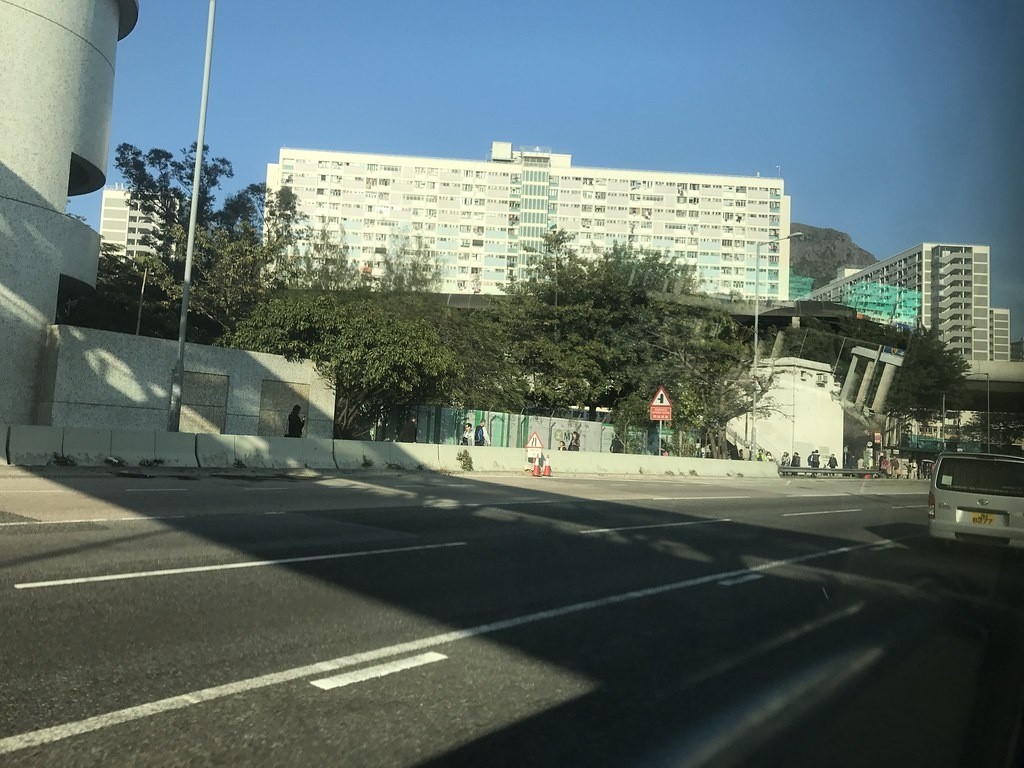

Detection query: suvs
[{"left": 925, "top": 450, "right": 1024, "bottom": 553}]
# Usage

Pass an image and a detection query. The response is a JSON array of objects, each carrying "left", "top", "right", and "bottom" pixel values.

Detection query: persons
[
  {"left": 401, "top": 415, "right": 417, "bottom": 443},
  {"left": 558, "top": 431, "right": 580, "bottom": 451},
  {"left": 460, "top": 419, "right": 491, "bottom": 446},
  {"left": 609, "top": 434, "right": 624, "bottom": 453},
  {"left": 285, "top": 405, "right": 306, "bottom": 438},
  {"left": 847, "top": 454, "right": 932, "bottom": 480},
  {"left": 730, "top": 445, "right": 838, "bottom": 477}
]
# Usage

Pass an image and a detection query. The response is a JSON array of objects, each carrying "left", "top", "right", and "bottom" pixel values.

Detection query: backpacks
[
  {"left": 475, "top": 425, "right": 484, "bottom": 442},
  {"left": 808, "top": 455, "right": 812, "bottom": 466},
  {"left": 813, "top": 455, "right": 819, "bottom": 463}
]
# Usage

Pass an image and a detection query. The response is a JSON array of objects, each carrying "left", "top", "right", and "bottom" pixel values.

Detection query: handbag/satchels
[{"left": 284, "top": 434, "right": 290, "bottom": 437}]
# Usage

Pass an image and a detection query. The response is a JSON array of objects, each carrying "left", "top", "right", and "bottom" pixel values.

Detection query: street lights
[
  {"left": 965, "top": 372, "right": 990, "bottom": 454},
  {"left": 746, "top": 231, "right": 804, "bottom": 461}
]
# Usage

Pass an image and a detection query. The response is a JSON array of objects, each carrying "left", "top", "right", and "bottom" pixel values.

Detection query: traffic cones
[
  {"left": 542, "top": 453, "right": 553, "bottom": 477},
  {"left": 531, "top": 453, "right": 542, "bottom": 477}
]
